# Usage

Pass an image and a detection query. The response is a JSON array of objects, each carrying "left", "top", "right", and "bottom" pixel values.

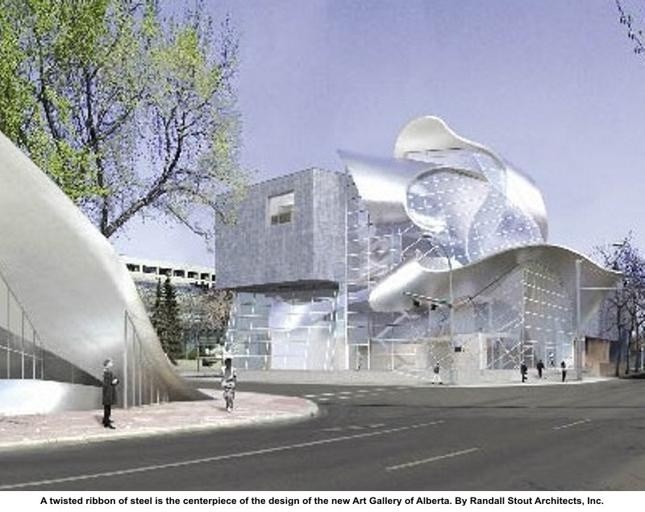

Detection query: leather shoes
[{"left": 103, "top": 420, "right": 115, "bottom": 429}]
